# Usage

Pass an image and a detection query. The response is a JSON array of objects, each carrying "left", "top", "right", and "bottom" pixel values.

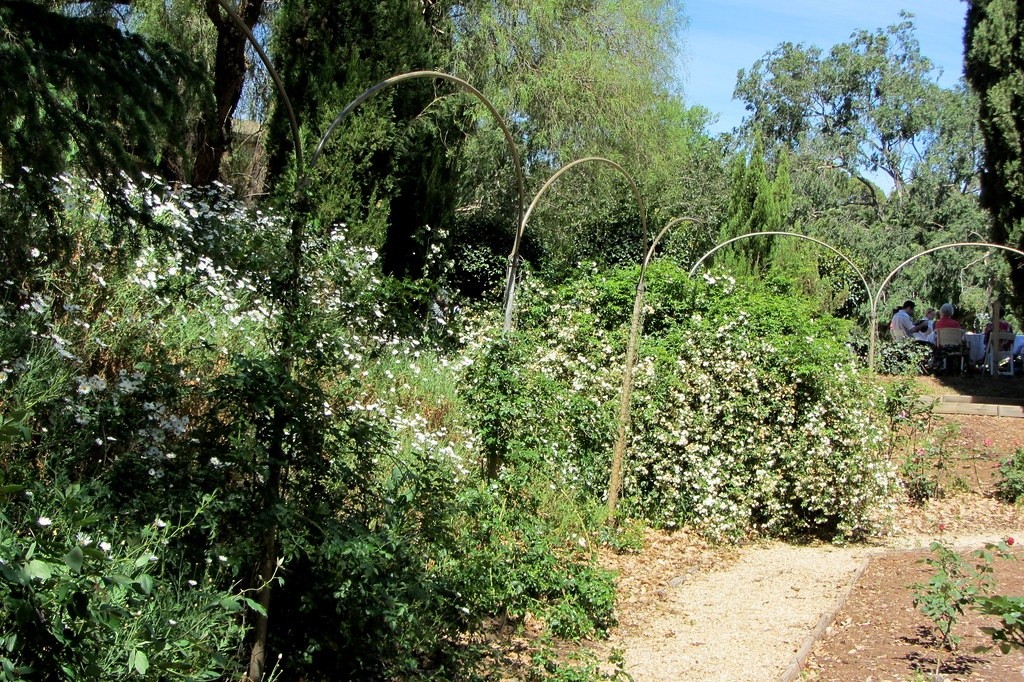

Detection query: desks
[{"left": 926, "top": 334, "right": 1024, "bottom": 371}]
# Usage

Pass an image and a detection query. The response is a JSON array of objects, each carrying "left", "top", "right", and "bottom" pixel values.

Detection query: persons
[{"left": 891, "top": 300, "right": 1014, "bottom": 376}]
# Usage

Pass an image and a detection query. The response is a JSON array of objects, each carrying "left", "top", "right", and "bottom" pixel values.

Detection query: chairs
[
  {"left": 890, "top": 326, "right": 909, "bottom": 342},
  {"left": 983, "top": 332, "right": 1016, "bottom": 376},
  {"left": 937, "top": 328, "right": 968, "bottom": 374}
]
